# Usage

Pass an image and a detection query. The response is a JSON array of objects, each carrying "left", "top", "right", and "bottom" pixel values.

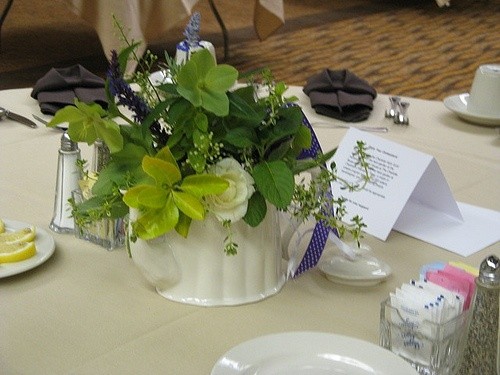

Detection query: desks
[{"left": 0, "top": 82, "right": 500, "bottom": 375}]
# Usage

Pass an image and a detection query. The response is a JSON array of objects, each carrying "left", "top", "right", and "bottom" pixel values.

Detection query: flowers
[{"left": 49, "top": 12, "right": 373, "bottom": 256}]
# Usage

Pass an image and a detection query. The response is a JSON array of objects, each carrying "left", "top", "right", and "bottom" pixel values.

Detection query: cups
[{"left": 467, "top": 65, "right": 500, "bottom": 113}]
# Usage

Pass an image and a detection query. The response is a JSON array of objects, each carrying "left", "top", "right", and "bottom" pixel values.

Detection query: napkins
[
  {"left": 31, "top": 66, "right": 111, "bottom": 119},
  {"left": 303, "top": 68, "right": 377, "bottom": 122}
]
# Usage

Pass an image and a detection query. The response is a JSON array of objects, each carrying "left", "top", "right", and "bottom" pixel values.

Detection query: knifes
[
  {"left": 310, "top": 121, "right": 387, "bottom": 132},
  {"left": 0, "top": 107, "right": 37, "bottom": 128}
]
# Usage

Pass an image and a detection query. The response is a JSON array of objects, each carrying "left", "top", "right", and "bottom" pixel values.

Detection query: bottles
[
  {"left": 49, "top": 131, "right": 83, "bottom": 232},
  {"left": 455, "top": 255, "right": 500, "bottom": 375}
]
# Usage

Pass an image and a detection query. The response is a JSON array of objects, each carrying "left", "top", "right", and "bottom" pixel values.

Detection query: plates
[
  {"left": 212, "top": 331, "right": 420, "bottom": 375},
  {"left": 0, "top": 218, "right": 55, "bottom": 278},
  {"left": 148, "top": 69, "right": 173, "bottom": 86},
  {"left": 444, "top": 93, "right": 500, "bottom": 125},
  {"left": 318, "top": 246, "right": 392, "bottom": 286}
]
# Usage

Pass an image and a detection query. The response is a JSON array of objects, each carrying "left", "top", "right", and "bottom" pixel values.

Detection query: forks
[{"left": 384, "top": 96, "right": 410, "bottom": 125}]
